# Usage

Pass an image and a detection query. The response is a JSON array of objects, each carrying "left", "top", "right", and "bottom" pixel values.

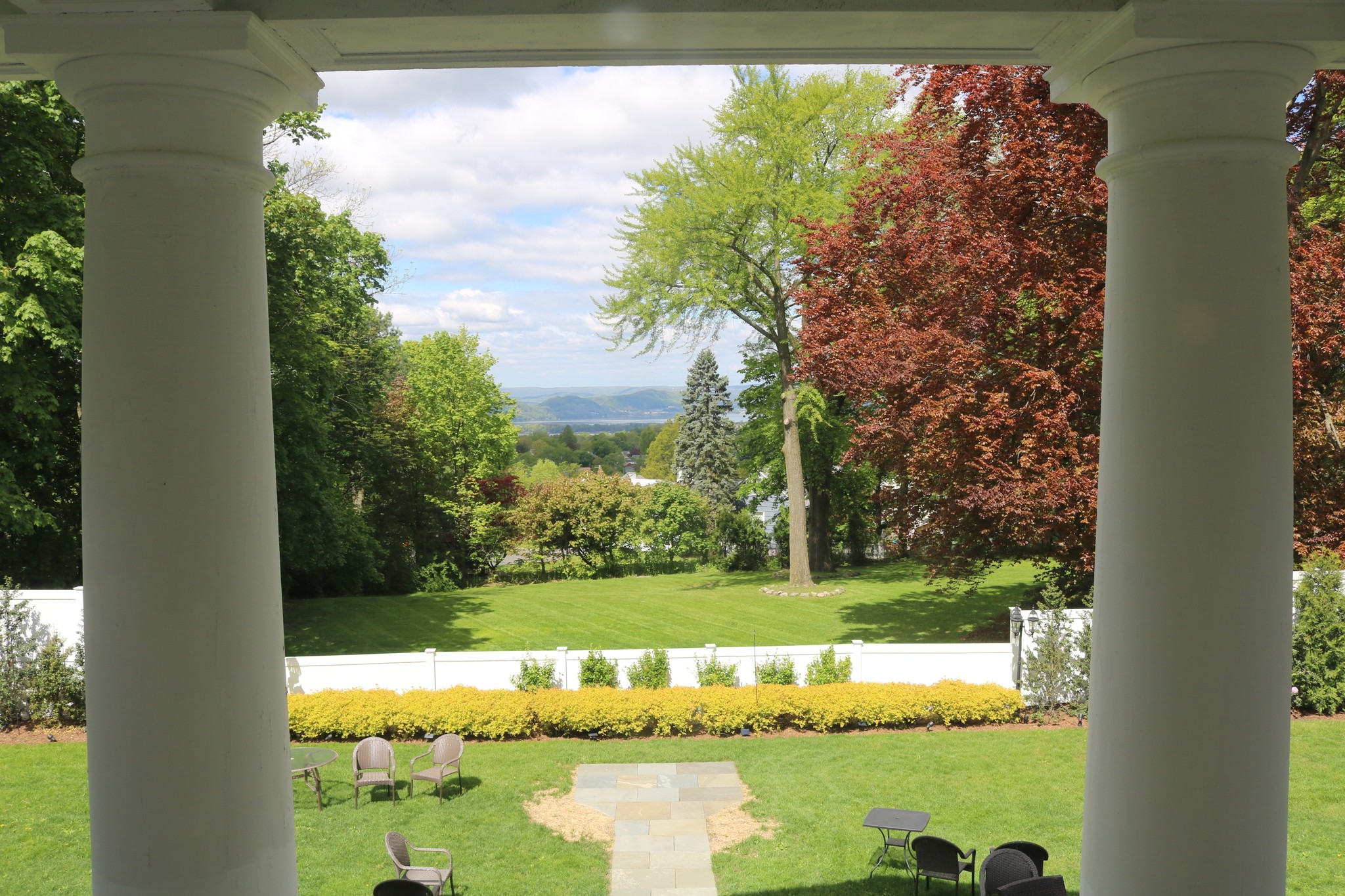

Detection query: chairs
[
  {"left": 997, "top": 874, "right": 1071, "bottom": 896},
  {"left": 410, "top": 733, "right": 465, "bottom": 805},
  {"left": 989, "top": 841, "right": 1049, "bottom": 877},
  {"left": 911, "top": 835, "right": 977, "bottom": 896},
  {"left": 373, "top": 879, "right": 441, "bottom": 896},
  {"left": 979, "top": 849, "right": 1039, "bottom": 896},
  {"left": 385, "top": 831, "right": 455, "bottom": 896},
  {"left": 352, "top": 736, "right": 396, "bottom": 809}
]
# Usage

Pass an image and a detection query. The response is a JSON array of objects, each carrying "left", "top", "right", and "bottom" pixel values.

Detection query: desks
[
  {"left": 862, "top": 806, "right": 931, "bottom": 883},
  {"left": 290, "top": 747, "right": 339, "bottom": 810}
]
